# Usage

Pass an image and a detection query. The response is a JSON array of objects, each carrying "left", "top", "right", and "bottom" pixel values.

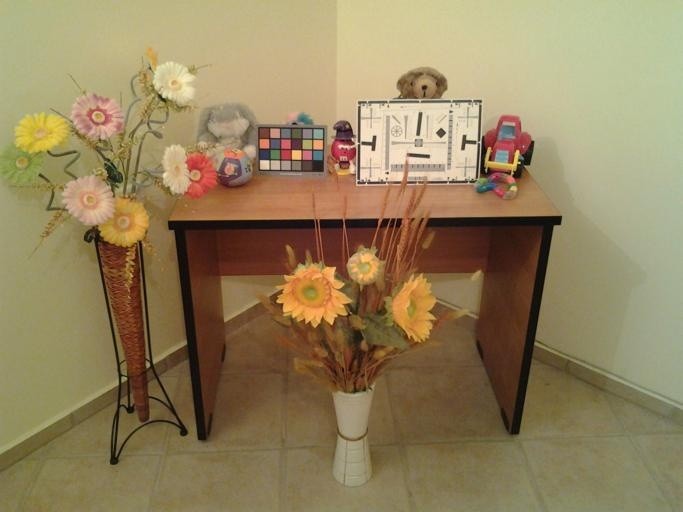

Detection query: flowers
[
  {"left": 0, "top": 40, "right": 216, "bottom": 251},
  {"left": 258, "top": 156, "right": 473, "bottom": 395}
]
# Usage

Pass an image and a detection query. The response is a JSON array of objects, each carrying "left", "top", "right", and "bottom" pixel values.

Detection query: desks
[{"left": 162, "top": 168, "right": 564, "bottom": 443}]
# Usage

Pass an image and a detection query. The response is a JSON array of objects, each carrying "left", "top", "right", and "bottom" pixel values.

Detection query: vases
[
  {"left": 93, "top": 236, "right": 188, "bottom": 466},
  {"left": 330, "top": 386, "right": 372, "bottom": 487}
]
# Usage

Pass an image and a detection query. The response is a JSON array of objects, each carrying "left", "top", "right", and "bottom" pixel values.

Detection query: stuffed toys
[
  {"left": 394, "top": 65, "right": 448, "bottom": 100},
  {"left": 192, "top": 102, "right": 259, "bottom": 187}
]
